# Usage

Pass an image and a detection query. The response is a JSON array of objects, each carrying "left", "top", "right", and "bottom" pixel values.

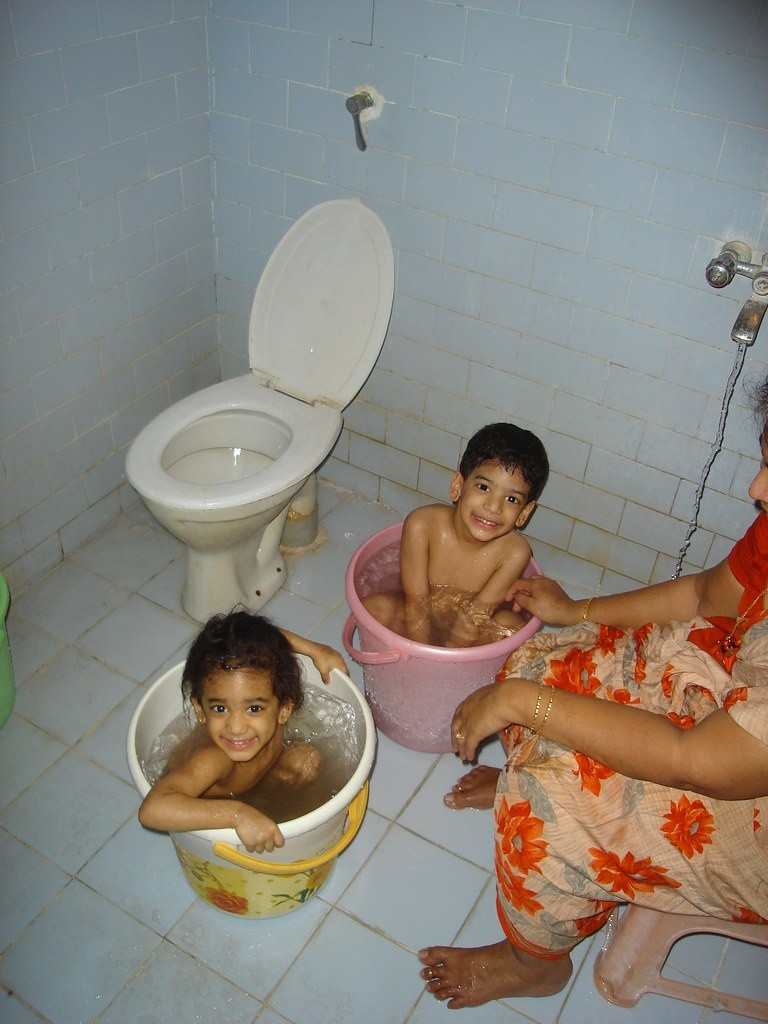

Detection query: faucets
[{"left": 730, "top": 296, "right": 766, "bottom": 345}]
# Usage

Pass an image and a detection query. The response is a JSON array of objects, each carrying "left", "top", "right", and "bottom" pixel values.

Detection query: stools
[{"left": 592, "top": 900, "right": 768, "bottom": 1022}]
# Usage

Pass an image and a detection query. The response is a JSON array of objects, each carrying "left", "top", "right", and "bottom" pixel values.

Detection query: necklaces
[{"left": 721, "top": 589, "right": 768, "bottom": 658}]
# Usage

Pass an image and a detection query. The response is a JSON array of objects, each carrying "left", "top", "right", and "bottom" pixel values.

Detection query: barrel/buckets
[
  {"left": 342, "top": 521, "right": 546, "bottom": 758},
  {"left": 124, "top": 658, "right": 375, "bottom": 920}
]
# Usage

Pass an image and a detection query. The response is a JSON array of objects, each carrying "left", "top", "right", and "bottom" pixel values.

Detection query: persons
[
  {"left": 137, "top": 613, "right": 351, "bottom": 854},
  {"left": 418, "top": 375, "right": 768, "bottom": 1010},
  {"left": 358, "top": 421, "right": 550, "bottom": 648}
]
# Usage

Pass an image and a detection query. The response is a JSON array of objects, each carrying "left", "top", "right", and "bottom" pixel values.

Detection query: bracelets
[
  {"left": 582, "top": 595, "right": 597, "bottom": 626},
  {"left": 531, "top": 681, "right": 555, "bottom": 737}
]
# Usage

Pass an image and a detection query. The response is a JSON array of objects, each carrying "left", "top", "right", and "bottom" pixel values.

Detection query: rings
[
  {"left": 455, "top": 730, "right": 468, "bottom": 739},
  {"left": 428, "top": 967, "right": 433, "bottom": 980},
  {"left": 458, "top": 782, "right": 465, "bottom": 792}
]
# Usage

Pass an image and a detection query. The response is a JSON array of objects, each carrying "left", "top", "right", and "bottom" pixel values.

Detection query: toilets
[{"left": 124, "top": 197, "right": 397, "bottom": 625}]
